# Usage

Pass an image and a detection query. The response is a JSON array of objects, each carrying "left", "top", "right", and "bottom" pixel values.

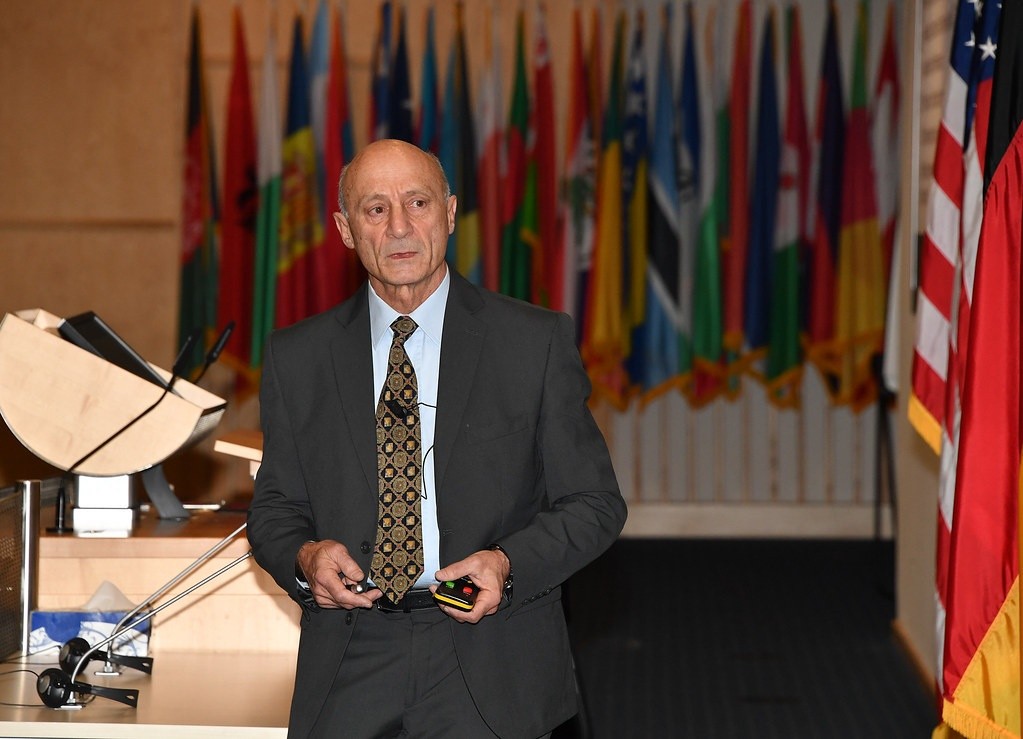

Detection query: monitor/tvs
[{"left": 59, "top": 311, "right": 176, "bottom": 394}]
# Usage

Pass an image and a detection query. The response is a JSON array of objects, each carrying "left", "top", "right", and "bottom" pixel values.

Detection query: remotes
[{"left": 433, "top": 577, "right": 480, "bottom": 611}]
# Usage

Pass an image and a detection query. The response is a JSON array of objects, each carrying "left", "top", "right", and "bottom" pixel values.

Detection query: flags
[
  {"left": 175, "top": 1, "right": 899, "bottom": 415},
  {"left": 907, "top": 0, "right": 1023, "bottom": 739}
]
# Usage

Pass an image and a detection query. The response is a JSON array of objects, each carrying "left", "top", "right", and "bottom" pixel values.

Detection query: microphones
[
  {"left": 61, "top": 550, "right": 254, "bottom": 709},
  {"left": 390, "top": 401, "right": 409, "bottom": 418},
  {"left": 46, "top": 318, "right": 237, "bottom": 534}
]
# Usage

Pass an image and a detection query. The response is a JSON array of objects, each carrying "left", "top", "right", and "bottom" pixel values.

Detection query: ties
[{"left": 368, "top": 316, "right": 425, "bottom": 605}]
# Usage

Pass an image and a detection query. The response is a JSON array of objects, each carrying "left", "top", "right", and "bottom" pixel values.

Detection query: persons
[{"left": 246, "top": 140, "right": 629, "bottom": 739}]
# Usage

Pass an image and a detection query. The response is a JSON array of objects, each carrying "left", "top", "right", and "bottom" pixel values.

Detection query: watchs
[{"left": 486, "top": 544, "right": 513, "bottom": 590}]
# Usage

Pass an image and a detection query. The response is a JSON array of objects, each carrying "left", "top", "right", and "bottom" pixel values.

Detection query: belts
[{"left": 364, "top": 587, "right": 439, "bottom": 612}]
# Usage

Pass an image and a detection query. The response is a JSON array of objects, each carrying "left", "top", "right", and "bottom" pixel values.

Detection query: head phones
[{"left": 37, "top": 636, "right": 153, "bottom": 708}]
